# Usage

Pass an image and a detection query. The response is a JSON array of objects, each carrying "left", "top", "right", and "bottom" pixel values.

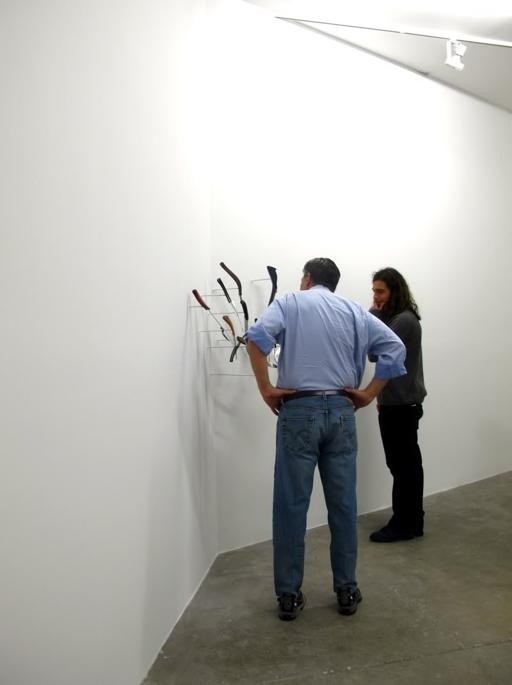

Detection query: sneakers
[
  {"left": 369, "top": 522, "right": 425, "bottom": 543},
  {"left": 337, "top": 587, "right": 362, "bottom": 614},
  {"left": 280, "top": 591, "right": 307, "bottom": 619}
]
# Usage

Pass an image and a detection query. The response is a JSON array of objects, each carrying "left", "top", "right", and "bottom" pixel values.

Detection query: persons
[
  {"left": 367, "top": 267, "right": 427, "bottom": 544},
  {"left": 244, "top": 257, "right": 407, "bottom": 622}
]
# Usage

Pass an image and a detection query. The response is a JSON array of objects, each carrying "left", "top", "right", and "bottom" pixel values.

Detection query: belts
[{"left": 278, "top": 390, "right": 354, "bottom": 407}]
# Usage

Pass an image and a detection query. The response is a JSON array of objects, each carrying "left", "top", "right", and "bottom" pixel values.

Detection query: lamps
[{"left": 440, "top": 38, "right": 467, "bottom": 73}]
